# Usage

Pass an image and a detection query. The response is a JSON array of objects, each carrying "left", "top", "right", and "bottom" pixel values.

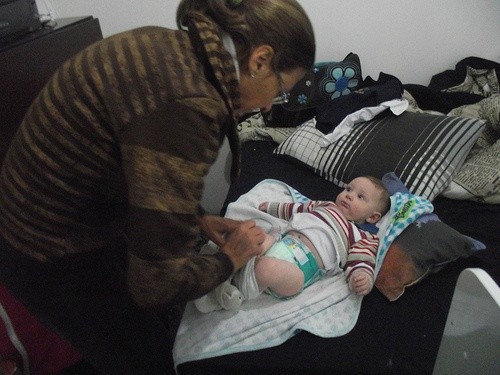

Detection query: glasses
[{"left": 271, "top": 70, "right": 290, "bottom": 104}]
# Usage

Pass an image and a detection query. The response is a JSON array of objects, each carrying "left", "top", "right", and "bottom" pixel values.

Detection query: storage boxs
[{"left": 0, "top": 0, "right": 42, "bottom": 45}]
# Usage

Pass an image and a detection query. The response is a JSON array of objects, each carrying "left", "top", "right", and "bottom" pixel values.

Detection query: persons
[
  {"left": 0, "top": 0, "right": 316, "bottom": 374},
  {"left": 193, "top": 174, "right": 393, "bottom": 317}
]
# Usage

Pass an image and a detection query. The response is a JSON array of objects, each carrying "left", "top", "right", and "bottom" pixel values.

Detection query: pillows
[
  {"left": 374, "top": 171, "right": 487, "bottom": 302},
  {"left": 273, "top": 111, "right": 487, "bottom": 203}
]
